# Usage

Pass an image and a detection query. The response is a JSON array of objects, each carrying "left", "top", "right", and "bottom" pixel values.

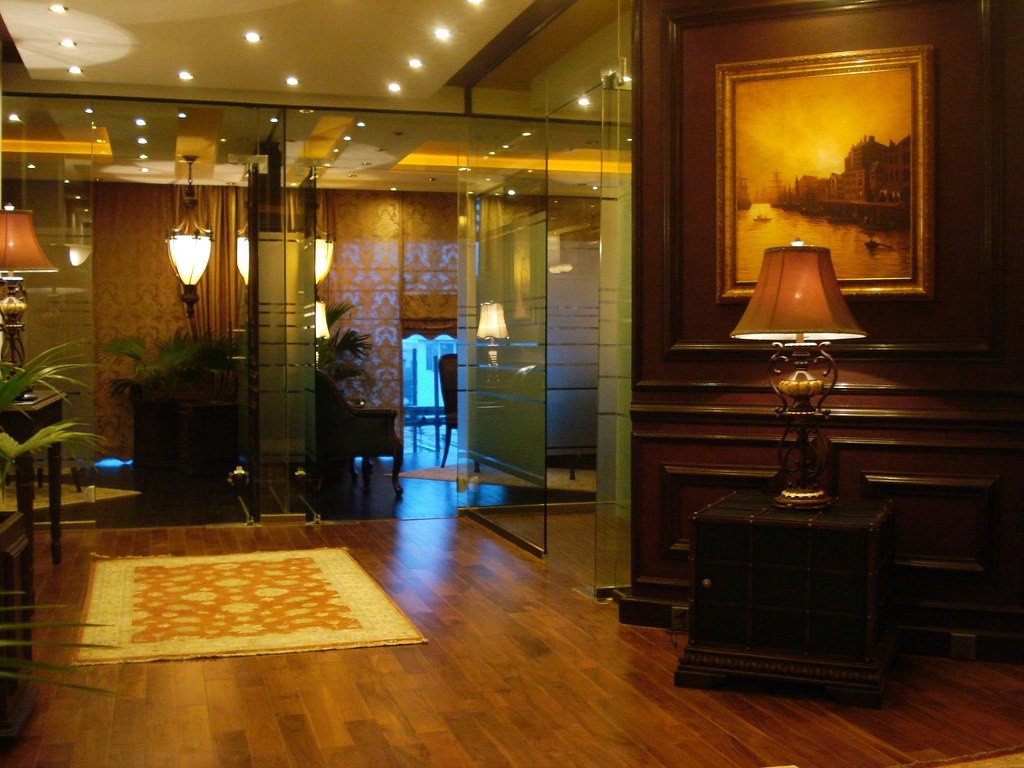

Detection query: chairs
[
  {"left": 316, "top": 369, "right": 405, "bottom": 497},
  {"left": 439, "top": 355, "right": 457, "bottom": 468}
]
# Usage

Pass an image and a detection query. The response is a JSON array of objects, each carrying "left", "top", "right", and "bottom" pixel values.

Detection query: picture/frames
[{"left": 714, "top": 45, "right": 936, "bottom": 305}]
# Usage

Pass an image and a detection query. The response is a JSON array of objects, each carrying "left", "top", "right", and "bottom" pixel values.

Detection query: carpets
[
  {"left": 0, "top": 481, "right": 140, "bottom": 512},
  {"left": 381, "top": 462, "right": 597, "bottom": 493},
  {"left": 69, "top": 545, "right": 430, "bottom": 667}
]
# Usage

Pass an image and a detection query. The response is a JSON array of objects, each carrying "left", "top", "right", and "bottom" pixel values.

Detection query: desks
[
  {"left": 0, "top": 390, "right": 69, "bottom": 617},
  {"left": 674, "top": 488, "right": 898, "bottom": 710}
]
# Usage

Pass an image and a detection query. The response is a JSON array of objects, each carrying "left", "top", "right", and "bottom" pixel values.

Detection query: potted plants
[
  {"left": 150, "top": 324, "right": 247, "bottom": 497},
  {"left": 96, "top": 337, "right": 178, "bottom": 471}
]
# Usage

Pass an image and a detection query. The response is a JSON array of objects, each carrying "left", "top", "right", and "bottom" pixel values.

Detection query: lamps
[
  {"left": 0, "top": 202, "right": 59, "bottom": 403},
  {"left": 315, "top": 227, "right": 334, "bottom": 303},
  {"left": 164, "top": 154, "right": 215, "bottom": 319},
  {"left": 315, "top": 301, "right": 330, "bottom": 370},
  {"left": 729, "top": 236, "right": 868, "bottom": 513},
  {"left": 236, "top": 223, "right": 250, "bottom": 286},
  {"left": 475, "top": 301, "right": 511, "bottom": 386}
]
